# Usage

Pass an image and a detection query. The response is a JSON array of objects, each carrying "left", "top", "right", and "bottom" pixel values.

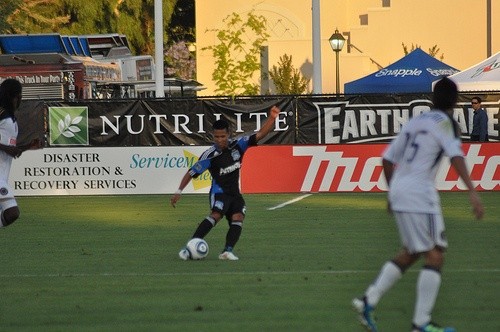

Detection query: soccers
[{"left": 185, "top": 238, "right": 210, "bottom": 260}]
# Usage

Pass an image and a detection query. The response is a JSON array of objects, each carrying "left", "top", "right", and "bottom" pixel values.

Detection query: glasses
[{"left": 471, "top": 102, "right": 478, "bottom": 104}]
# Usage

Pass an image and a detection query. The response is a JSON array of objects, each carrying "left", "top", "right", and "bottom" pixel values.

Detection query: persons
[
  {"left": 470, "top": 97, "right": 489, "bottom": 142},
  {"left": 0, "top": 78, "right": 23, "bottom": 229},
  {"left": 351, "top": 78, "right": 484, "bottom": 332},
  {"left": 169, "top": 106, "right": 281, "bottom": 261}
]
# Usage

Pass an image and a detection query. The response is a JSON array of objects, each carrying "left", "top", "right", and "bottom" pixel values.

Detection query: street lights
[{"left": 328, "top": 28, "right": 345, "bottom": 97}]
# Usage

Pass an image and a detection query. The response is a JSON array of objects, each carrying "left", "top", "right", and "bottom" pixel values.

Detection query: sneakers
[
  {"left": 178, "top": 248, "right": 190, "bottom": 261},
  {"left": 218, "top": 251, "right": 239, "bottom": 260},
  {"left": 352, "top": 295, "right": 377, "bottom": 331},
  {"left": 411, "top": 320, "right": 456, "bottom": 331}
]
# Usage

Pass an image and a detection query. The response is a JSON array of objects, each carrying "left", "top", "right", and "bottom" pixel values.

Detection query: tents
[
  {"left": 344, "top": 48, "right": 462, "bottom": 94},
  {"left": 432, "top": 52, "right": 500, "bottom": 93}
]
